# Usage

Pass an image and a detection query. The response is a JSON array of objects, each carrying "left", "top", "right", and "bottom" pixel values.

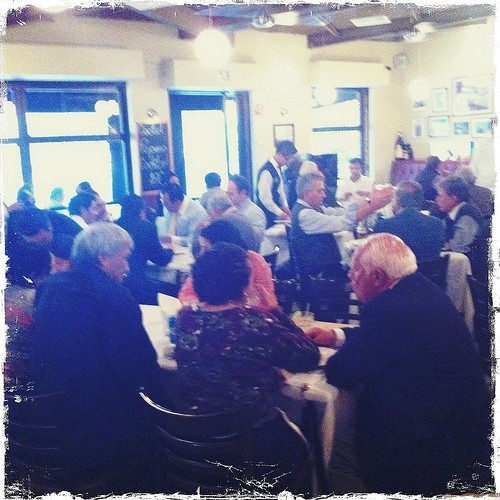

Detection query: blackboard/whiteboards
[{"left": 138, "top": 123, "right": 171, "bottom": 192}]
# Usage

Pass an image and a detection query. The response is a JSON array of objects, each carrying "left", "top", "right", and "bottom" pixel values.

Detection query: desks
[
  {"left": 146, "top": 242, "right": 195, "bottom": 286},
  {"left": 139, "top": 305, "right": 354, "bottom": 493}
]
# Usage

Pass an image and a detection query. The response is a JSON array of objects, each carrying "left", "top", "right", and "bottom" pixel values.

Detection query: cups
[
  {"left": 292, "top": 310, "right": 315, "bottom": 334},
  {"left": 181, "top": 297, "right": 198, "bottom": 311}
]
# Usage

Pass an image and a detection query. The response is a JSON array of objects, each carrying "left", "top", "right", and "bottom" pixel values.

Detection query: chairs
[
  {"left": 8, "top": 391, "right": 120, "bottom": 496},
  {"left": 135, "top": 386, "right": 310, "bottom": 494},
  {"left": 417, "top": 254, "right": 449, "bottom": 285},
  {"left": 304, "top": 275, "right": 361, "bottom": 321},
  {"left": 467, "top": 274, "right": 490, "bottom": 341},
  {"left": 274, "top": 278, "right": 296, "bottom": 314},
  {"left": 262, "top": 244, "right": 280, "bottom": 278}
]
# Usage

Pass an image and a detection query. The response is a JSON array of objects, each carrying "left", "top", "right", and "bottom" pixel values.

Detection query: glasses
[{"left": 283, "top": 154, "right": 290, "bottom": 164}]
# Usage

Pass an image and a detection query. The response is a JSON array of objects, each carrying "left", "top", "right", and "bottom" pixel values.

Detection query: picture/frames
[
  {"left": 428, "top": 116, "right": 450, "bottom": 137},
  {"left": 471, "top": 118, "right": 491, "bottom": 138},
  {"left": 450, "top": 73, "right": 494, "bottom": 116},
  {"left": 432, "top": 89, "right": 448, "bottom": 112}
]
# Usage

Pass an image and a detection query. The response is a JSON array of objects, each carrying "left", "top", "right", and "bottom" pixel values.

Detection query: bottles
[
  {"left": 404, "top": 143, "right": 413, "bottom": 160},
  {"left": 394, "top": 136, "right": 404, "bottom": 160}
]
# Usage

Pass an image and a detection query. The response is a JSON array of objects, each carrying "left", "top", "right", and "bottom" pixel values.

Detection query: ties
[{"left": 169, "top": 213, "right": 176, "bottom": 236}]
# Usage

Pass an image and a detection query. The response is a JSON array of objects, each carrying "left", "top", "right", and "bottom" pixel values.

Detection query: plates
[{"left": 317, "top": 346, "right": 337, "bottom": 366}]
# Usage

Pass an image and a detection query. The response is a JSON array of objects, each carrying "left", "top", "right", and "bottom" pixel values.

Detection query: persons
[
  {"left": 26, "top": 220, "right": 173, "bottom": 493},
  {"left": 168, "top": 241, "right": 323, "bottom": 496},
  {"left": 177, "top": 218, "right": 277, "bottom": 312},
  {"left": 4, "top": 140, "right": 494, "bottom": 391},
  {"left": 303, "top": 232, "right": 487, "bottom": 496}
]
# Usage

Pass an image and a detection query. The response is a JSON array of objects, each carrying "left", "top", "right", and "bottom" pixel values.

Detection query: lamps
[{"left": 252, "top": 6, "right": 274, "bottom": 28}]
